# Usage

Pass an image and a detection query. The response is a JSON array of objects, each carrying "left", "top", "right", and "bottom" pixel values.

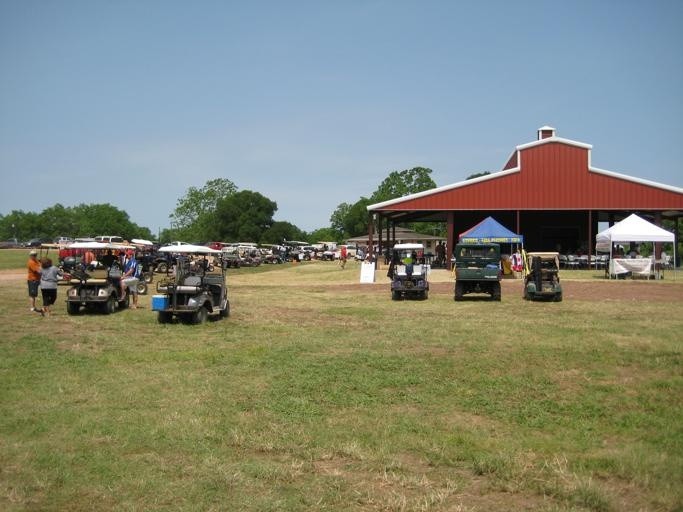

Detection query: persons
[
  {"left": 101, "top": 250, "right": 118, "bottom": 267},
  {"left": 339, "top": 247, "right": 346, "bottom": 267},
  {"left": 441, "top": 242, "right": 447, "bottom": 266},
  {"left": 25, "top": 251, "right": 42, "bottom": 312},
  {"left": 434, "top": 241, "right": 443, "bottom": 267},
  {"left": 38, "top": 256, "right": 65, "bottom": 317},
  {"left": 116, "top": 250, "right": 140, "bottom": 310},
  {"left": 79, "top": 248, "right": 95, "bottom": 265}
]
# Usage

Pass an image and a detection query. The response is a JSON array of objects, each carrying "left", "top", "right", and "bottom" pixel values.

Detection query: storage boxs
[{"left": 151, "top": 294, "right": 170, "bottom": 309}]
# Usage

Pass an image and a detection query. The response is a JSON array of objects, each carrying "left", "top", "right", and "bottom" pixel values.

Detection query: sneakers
[{"left": 31, "top": 307, "right": 51, "bottom": 316}]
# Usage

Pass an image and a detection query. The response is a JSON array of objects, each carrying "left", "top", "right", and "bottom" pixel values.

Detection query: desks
[
  {"left": 424, "top": 254, "right": 434, "bottom": 264},
  {"left": 612, "top": 259, "right": 651, "bottom": 281}
]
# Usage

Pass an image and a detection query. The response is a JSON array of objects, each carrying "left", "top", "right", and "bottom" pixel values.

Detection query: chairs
[
  {"left": 395, "top": 265, "right": 407, "bottom": 276},
  {"left": 184, "top": 276, "right": 201, "bottom": 287},
  {"left": 94, "top": 269, "right": 108, "bottom": 279},
  {"left": 411, "top": 265, "right": 422, "bottom": 275},
  {"left": 561, "top": 252, "right": 672, "bottom": 271}
]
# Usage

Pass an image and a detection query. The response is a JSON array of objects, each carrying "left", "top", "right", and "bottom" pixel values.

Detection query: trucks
[{"left": 452, "top": 242, "right": 503, "bottom": 301}]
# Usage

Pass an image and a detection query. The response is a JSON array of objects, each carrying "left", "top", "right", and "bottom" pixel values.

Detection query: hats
[
  {"left": 125, "top": 250, "right": 133, "bottom": 254},
  {"left": 29, "top": 251, "right": 37, "bottom": 255}
]
